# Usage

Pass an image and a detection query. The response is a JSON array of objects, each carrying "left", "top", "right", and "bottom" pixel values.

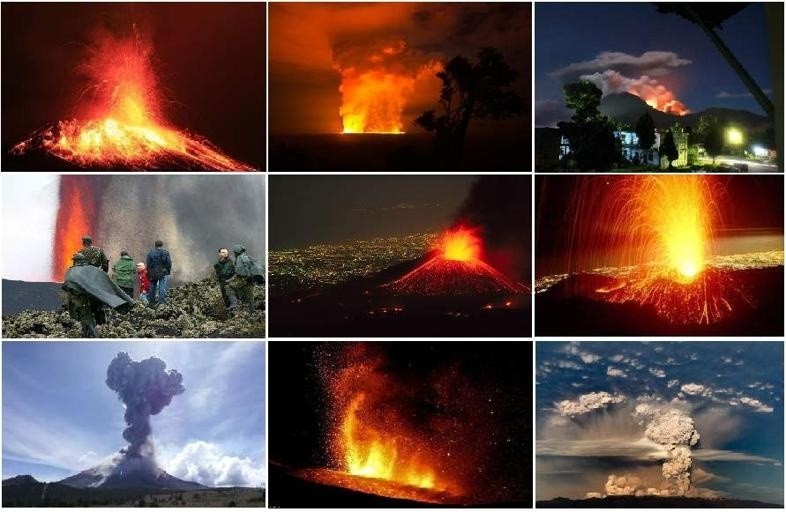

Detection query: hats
[
  {"left": 82, "top": 236, "right": 92, "bottom": 243},
  {"left": 136, "top": 262, "right": 145, "bottom": 270},
  {"left": 72, "top": 253, "right": 87, "bottom": 260}
]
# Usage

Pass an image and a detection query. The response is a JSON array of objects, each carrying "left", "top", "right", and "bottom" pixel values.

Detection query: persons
[
  {"left": 136, "top": 262, "right": 151, "bottom": 306},
  {"left": 112, "top": 251, "right": 136, "bottom": 314},
  {"left": 225, "top": 245, "right": 265, "bottom": 314},
  {"left": 214, "top": 248, "right": 235, "bottom": 308},
  {"left": 62, "top": 253, "right": 97, "bottom": 338},
  {"left": 146, "top": 240, "right": 172, "bottom": 305},
  {"left": 78, "top": 235, "right": 108, "bottom": 324}
]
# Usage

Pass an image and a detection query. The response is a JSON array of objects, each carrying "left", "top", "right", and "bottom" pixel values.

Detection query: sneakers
[{"left": 225, "top": 304, "right": 238, "bottom": 311}]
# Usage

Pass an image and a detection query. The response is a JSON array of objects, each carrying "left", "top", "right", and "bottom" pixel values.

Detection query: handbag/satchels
[{"left": 147, "top": 268, "right": 162, "bottom": 282}]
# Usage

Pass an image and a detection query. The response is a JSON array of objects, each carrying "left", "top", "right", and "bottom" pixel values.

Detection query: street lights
[{"left": 729, "top": 130, "right": 742, "bottom": 161}]
